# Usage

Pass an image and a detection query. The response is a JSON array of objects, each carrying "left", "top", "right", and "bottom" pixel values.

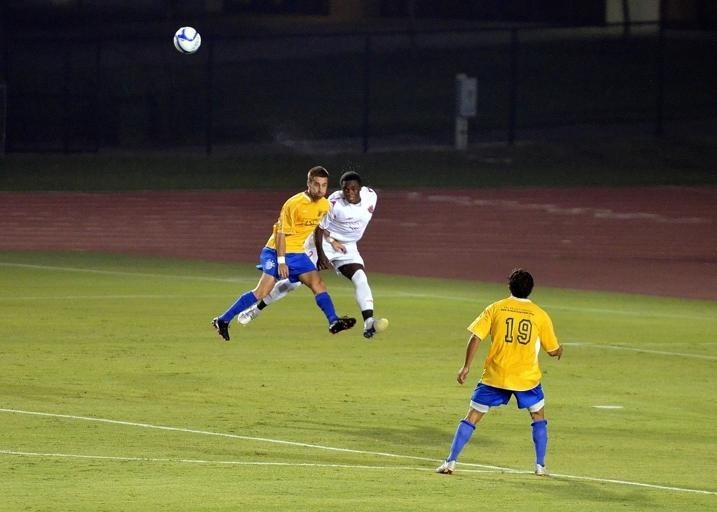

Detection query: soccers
[{"left": 173, "top": 27, "right": 201, "bottom": 54}]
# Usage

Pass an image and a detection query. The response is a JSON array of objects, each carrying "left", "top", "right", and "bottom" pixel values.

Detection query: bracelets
[
  {"left": 277, "top": 256, "right": 286, "bottom": 264},
  {"left": 326, "top": 238, "right": 333, "bottom": 244}
]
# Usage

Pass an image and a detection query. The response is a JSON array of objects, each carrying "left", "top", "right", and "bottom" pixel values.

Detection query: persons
[
  {"left": 435, "top": 271, "right": 564, "bottom": 476},
  {"left": 238, "top": 171, "right": 389, "bottom": 339},
  {"left": 212, "top": 165, "right": 357, "bottom": 341}
]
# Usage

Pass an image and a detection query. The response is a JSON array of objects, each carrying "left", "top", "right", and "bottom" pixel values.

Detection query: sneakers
[
  {"left": 535, "top": 464, "right": 546, "bottom": 476},
  {"left": 435, "top": 460, "right": 456, "bottom": 473},
  {"left": 363, "top": 318, "right": 389, "bottom": 338},
  {"left": 329, "top": 316, "right": 356, "bottom": 334},
  {"left": 238, "top": 303, "right": 261, "bottom": 325},
  {"left": 210, "top": 316, "right": 230, "bottom": 341}
]
